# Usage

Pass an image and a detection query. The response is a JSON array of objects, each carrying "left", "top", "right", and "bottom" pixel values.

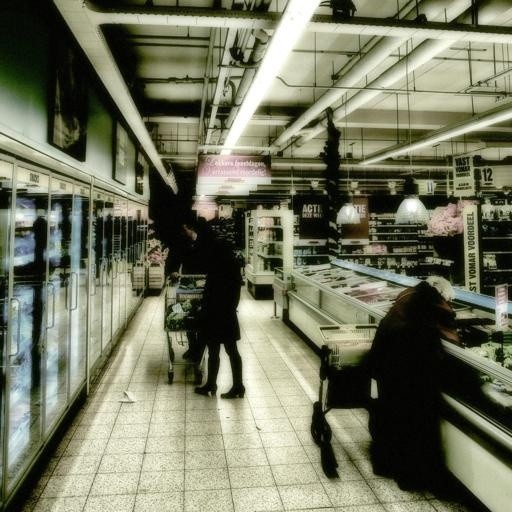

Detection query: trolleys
[
  {"left": 163, "top": 275, "right": 206, "bottom": 385},
  {"left": 310, "top": 324, "right": 380, "bottom": 477}
]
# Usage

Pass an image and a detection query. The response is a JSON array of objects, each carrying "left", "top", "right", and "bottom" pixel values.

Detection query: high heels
[
  {"left": 221, "top": 384, "right": 245, "bottom": 398},
  {"left": 195, "top": 383, "right": 217, "bottom": 395}
]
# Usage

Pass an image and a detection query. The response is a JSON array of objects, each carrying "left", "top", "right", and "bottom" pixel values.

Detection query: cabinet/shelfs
[
  {"left": 246, "top": 189, "right": 511, "bottom": 300},
  {"left": 271, "top": 258, "right": 510, "bottom": 512}
]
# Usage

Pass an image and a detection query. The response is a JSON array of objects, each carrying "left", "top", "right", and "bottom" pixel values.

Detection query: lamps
[
  {"left": 336, "top": 40, "right": 361, "bottom": 225},
  {"left": 358, "top": 102, "right": 512, "bottom": 165},
  {"left": 393, "top": 41, "right": 428, "bottom": 226},
  {"left": 220, "top": 0, "right": 323, "bottom": 156}
]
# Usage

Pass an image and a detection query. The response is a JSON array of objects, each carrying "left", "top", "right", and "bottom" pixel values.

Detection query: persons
[
  {"left": 180, "top": 213, "right": 246, "bottom": 400},
  {"left": 365, "top": 273, "right": 463, "bottom": 500},
  {"left": 164, "top": 221, "right": 212, "bottom": 364}
]
